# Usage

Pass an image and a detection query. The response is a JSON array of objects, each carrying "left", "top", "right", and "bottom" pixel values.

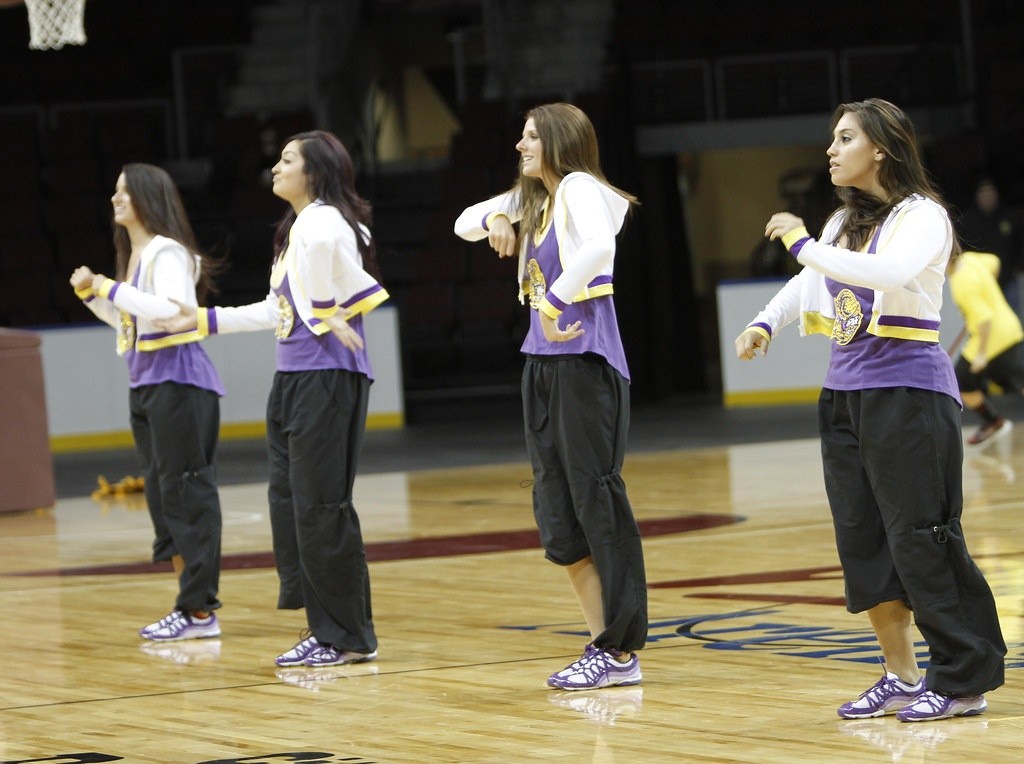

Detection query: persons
[
  {"left": 69, "top": 164, "right": 225, "bottom": 644},
  {"left": 954, "top": 182, "right": 1024, "bottom": 443},
  {"left": 455, "top": 103, "right": 649, "bottom": 691},
  {"left": 152, "top": 132, "right": 390, "bottom": 668},
  {"left": 734, "top": 98, "right": 1010, "bottom": 726}
]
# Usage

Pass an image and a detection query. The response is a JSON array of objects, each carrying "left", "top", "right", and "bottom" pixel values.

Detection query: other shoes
[{"left": 968, "top": 418, "right": 1010, "bottom": 451}]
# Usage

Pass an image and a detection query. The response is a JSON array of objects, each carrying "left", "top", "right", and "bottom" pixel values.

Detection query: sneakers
[
  {"left": 839, "top": 718, "right": 988, "bottom": 761},
  {"left": 274, "top": 636, "right": 378, "bottom": 667},
  {"left": 838, "top": 654, "right": 924, "bottom": 717},
  {"left": 139, "top": 610, "right": 221, "bottom": 641},
  {"left": 897, "top": 687, "right": 987, "bottom": 722},
  {"left": 548, "top": 689, "right": 643, "bottom": 726},
  {"left": 547, "top": 642, "right": 642, "bottom": 691},
  {"left": 274, "top": 666, "right": 378, "bottom": 692},
  {"left": 140, "top": 641, "right": 221, "bottom": 665}
]
